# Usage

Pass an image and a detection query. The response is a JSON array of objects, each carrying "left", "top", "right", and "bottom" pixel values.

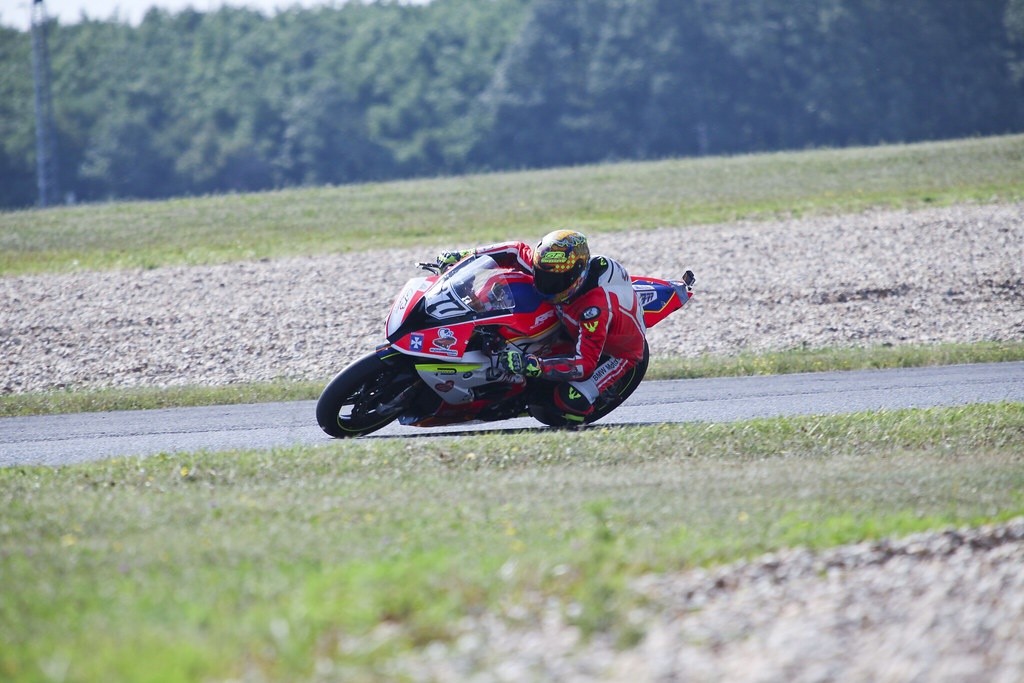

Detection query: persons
[{"left": 437, "top": 230, "right": 650, "bottom": 424}]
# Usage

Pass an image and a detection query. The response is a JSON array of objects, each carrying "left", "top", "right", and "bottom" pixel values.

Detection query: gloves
[
  {"left": 500, "top": 351, "right": 543, "bottom": 378},
  {"left": 437, "top": 248, "right": 475, "bottom": 273}
]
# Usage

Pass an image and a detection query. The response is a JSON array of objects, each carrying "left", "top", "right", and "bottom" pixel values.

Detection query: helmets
[{"left": 532, "top": 228, "right": 589, "bottom": 305}]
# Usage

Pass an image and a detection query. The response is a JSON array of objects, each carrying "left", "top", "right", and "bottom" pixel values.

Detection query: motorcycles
[{"left": 313, "top": 256, "right": 696, "bottom": 440}]
